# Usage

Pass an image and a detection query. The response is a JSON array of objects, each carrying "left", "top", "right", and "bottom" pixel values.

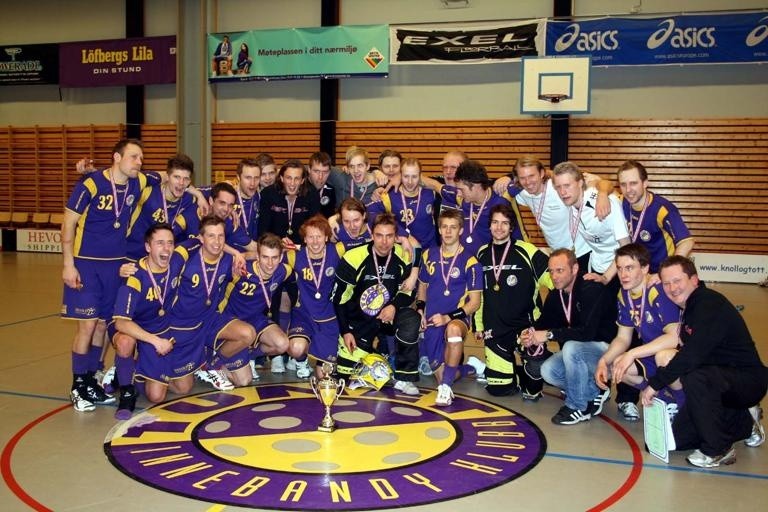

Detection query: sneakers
[
  {"left": 589, "top": 383, "right": 610, "bottom": 417},
  {"left": 742, "top": 401, "right": 766, "bottom": 448},
  {"left": 618, "top": 402, "right": 641, "bottom": 421},
  {"left": 270, "top": 356, "right": 315, "bottom": 379},
  {"left": 552, "top": 402, "right": 591, "bottom": 425},
  {"left": 393, "top": 379, "right": 420, "bottom": 396},
  {"left": 193, "top": 363, "right": 235, "bottom": 391},
  {"left": 115, "top": 387, "right": 137, "bottom": 421},
  {"left": 247, "top": 359, "right": 260, "bottom": 379},
  {"left": 72, "top": 365, "right": 116, "bottom": 405},
  {"left": 435, "top": 383, "right": 455, "bottom": 407},
  {"left": 70, "top": 384, "right": 95, "bottom": 411},
  {"left": 685, "top": 442, "right": 737, "bottom": 469},
  {"left": 466, "top": 355, "right": 487, "bottom": 379}
]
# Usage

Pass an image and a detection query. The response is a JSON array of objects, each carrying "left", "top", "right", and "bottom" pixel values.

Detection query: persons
[
  {"left": 211, "top": 35, "right": 234, "bottom": 78},
  {"left": 237, "top": 43, "right": 251, "bottom": 71}
]
